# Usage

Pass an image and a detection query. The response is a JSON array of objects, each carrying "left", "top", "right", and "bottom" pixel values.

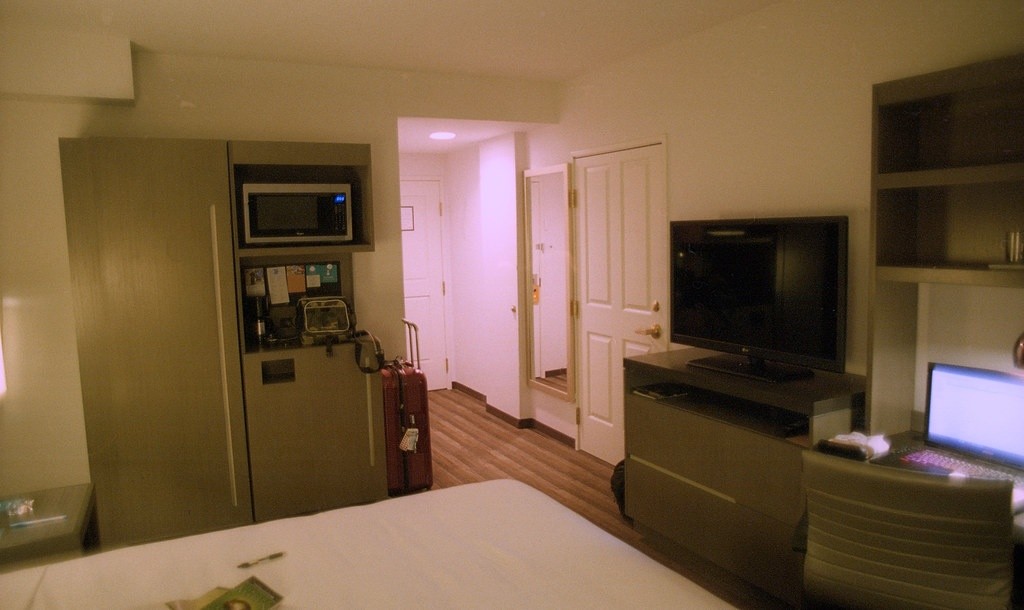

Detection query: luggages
[{"left": 381, "top": 354, "right": 433, "bottom": 496}]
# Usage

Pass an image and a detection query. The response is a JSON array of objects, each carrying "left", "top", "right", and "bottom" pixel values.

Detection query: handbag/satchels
[{"left": 295, "top": 296, "right": 357, "bottom": 358}]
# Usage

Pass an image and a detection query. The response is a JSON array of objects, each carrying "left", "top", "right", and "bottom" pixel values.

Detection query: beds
[{"left": 0, "top": 478, "right": 739, "bottom": 610}]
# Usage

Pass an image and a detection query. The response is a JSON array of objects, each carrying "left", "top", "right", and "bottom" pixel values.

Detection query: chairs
[{"left": 799, "top": 450, "right": 1014, "bottom": 610}]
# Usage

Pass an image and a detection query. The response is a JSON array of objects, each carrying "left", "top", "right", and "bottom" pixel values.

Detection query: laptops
[{"left": 868, "top": 362, "right": 1024, "bottom": 515}]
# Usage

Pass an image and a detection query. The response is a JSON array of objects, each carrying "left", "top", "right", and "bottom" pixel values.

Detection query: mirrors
[{"left": 524, "top": 163, "right": 576, "bottom": 405}]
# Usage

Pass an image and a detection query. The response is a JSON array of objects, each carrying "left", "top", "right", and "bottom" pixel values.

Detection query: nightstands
[{"left": 0, "top": 482, "right": 96, "bottom": 575}]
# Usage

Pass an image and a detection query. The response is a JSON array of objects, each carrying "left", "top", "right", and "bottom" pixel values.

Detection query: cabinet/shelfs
[
  {"left": 622, "top": 348, "right": 867, "bottom": 610},
  {"left": 863, "top": 54, "right": 1024, "bottom": 437}
]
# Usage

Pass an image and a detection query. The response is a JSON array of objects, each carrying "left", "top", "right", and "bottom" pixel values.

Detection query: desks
[{"left": 869, "top": 429, "right": 1024, "bottom": 545}]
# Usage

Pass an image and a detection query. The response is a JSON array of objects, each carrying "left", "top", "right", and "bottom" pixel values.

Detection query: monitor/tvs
[{"left": 672, "top": 216, "right": 851, "bottom": 383}]
